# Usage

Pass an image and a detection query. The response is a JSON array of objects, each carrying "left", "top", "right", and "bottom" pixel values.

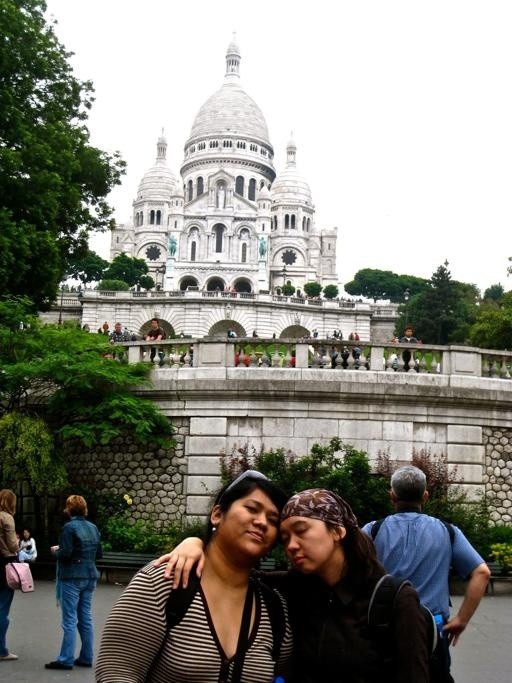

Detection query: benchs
[
  {"left": 93, "top": 548, "right": 163, "bottom": 586},
  {"left": 484, "top": 561, "right": 511, "bottom": 594}
]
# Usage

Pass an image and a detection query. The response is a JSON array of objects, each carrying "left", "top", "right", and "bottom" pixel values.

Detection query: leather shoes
[
  {"left": 45, "top": 662, "right": 73, "bottom": 670},
  {"left": 0, "top": 653, "right": 18, "bottom": 660},
  {"left": 74, "top": 660, "right": 93, "bottom": 668}
]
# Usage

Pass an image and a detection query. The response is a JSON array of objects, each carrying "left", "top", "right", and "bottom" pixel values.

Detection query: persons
[
  {"left": 154, "top": 488, "right": 438, "bottom": 681},
  {"left": 83, "top": 319, "right": 166, "bottom": 365},
  {"left": 330, "top": 331, "right": 360, "bottom": 369},
  {"left": 401, "top": 326, "right": 420, "bottom": 372},
  {"left": 0, "top": 488, "right": 38, "bottom": 659},
  {"left": 361, "top": 464, "right": 491, "bottom": 680},
  {"left": 44, "top": 494, "right": 101, "bottom": 670},
  {"left": 95, "top": 469, "right": 293, "bottom": 683}
]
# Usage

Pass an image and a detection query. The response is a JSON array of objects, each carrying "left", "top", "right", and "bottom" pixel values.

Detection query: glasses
[{"left": 217, "top": 468, "right": 273, "bottom": 504}]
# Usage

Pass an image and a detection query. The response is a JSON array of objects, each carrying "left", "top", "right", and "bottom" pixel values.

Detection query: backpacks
[{"left": 367, "top": 574, "right": 455, "bottom": 683}]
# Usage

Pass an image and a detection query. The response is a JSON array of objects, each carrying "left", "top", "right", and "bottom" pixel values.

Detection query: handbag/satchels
[{"left": 4, "top": 562, "right": 35, "bottom": 593}]
